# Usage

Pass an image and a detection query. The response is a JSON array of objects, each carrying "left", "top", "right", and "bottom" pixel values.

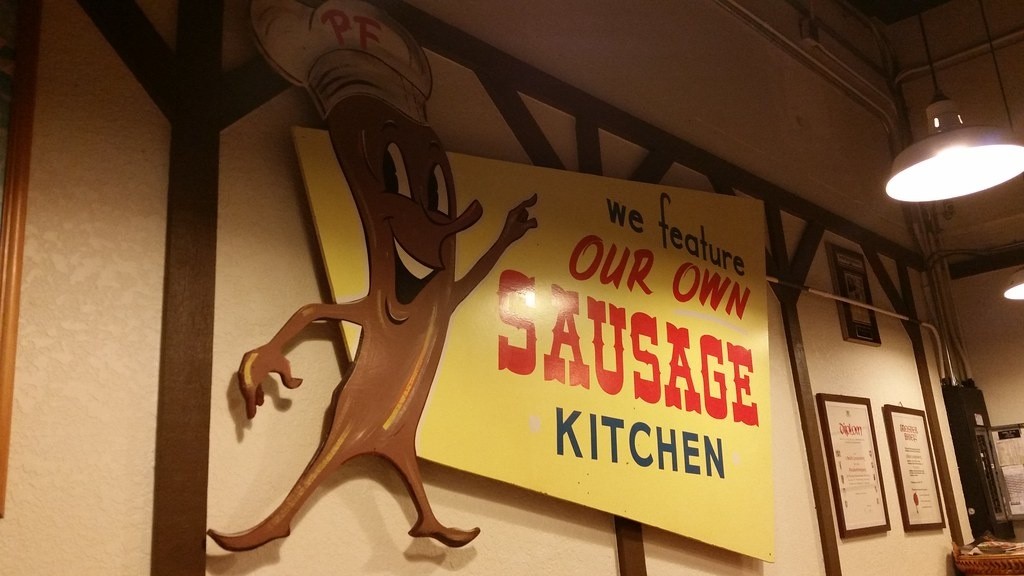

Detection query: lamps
[
  {"left": 883, "top": 14, "right": 1024, "bottom": 203},
  {"left": 1003, "top": 269, "right": 1024, "bottom": 301}
]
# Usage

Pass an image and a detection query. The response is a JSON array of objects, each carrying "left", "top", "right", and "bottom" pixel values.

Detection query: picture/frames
[
  {"left": 815, "top": 393, "right": 891, "bottom": 538},
  {"left": 826, "top": 241, "right": 882, "bottom": 347},
  {"left": 884, "top": 403, "right": 946, "bottom": 532}
]
